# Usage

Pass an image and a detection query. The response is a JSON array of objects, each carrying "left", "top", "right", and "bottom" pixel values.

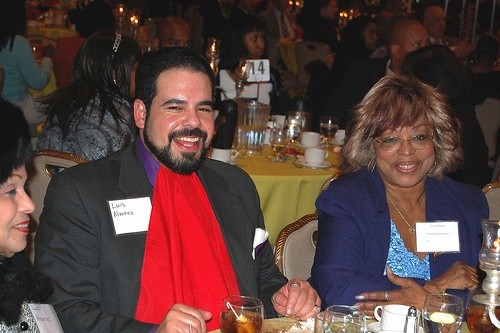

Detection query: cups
[
  {"left": 268, "top": 114, "right": 328, "bottom": 164},
  {"left": 220, "top": 295, "right": 264, "bottom": 333},
  {"left": 467, "top": 289, "right": 500, "bottom": 333},
  {"left": 211, "top": 146, "right": 238, "bottom": 162},
  {"left": 374, "top": 304, "right": 421, "bottom": 332},
  {"left": 422, "top": 292, "right": 464, "bottom": 333},
  {"left": 315, "top": 304, "right": 366, "bottom": 333}
]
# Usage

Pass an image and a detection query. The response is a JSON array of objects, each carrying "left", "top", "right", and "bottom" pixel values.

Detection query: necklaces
[{"left": 386, "top": 190, "right": 425, "bottom": 234}]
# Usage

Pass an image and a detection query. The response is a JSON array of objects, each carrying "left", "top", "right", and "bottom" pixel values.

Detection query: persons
[{"left": 0, "top": 0, "right": 500, "bottom": 333}]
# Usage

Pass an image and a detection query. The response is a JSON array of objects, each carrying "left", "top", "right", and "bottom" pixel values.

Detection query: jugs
[
  {"left": 286, "top": 95, "right": 313, "bottom": 139},
  {"left": 237, "top": 99, "right": 271, "bottom": 156}
]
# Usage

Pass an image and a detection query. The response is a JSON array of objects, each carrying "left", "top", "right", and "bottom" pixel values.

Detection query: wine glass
[
  {"left": 269, "top": 129, "right": 287, "bottom": 163},
  {"left": 320, "top": 116, "right": 339, "bottom": 145}
]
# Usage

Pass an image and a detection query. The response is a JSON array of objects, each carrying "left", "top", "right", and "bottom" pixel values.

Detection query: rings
[
  {"left": 290, "top": 280, "right": 301, "bottom": 287},
  {"left": 315, "top": 305, "right": 323, "bottom": 311},
  {"left": 385, "top": 291, "right": 389, "bottom": 302}
]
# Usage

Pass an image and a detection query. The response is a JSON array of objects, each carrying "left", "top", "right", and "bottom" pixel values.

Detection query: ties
[{"left": 433, "top": 39, "right": 441, "bottom": 45}]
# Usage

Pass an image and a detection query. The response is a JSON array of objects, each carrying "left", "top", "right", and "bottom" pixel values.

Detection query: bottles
[{"left": 404, "top": 305, "right": 418, "bottom": 332}]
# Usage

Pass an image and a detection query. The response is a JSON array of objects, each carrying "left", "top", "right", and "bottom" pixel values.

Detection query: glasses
[{"left": 373, "top": 131, "right": 437, "bottom": 150}]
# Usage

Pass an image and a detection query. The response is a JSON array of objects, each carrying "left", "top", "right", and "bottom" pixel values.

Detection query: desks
[
  {"left": 236, "top": 126, "right": 347, "bottom": 252},
  {"left": 474, "top": 97, "right": 500, "bottom": 182},
  {"left": 26, "top": 28, "right": 77, "bottom": 99}
]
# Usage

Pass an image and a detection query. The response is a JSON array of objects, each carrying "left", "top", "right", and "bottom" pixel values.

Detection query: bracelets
[{"left": 487, "top": 182, "right": 493, "bottom": 190}]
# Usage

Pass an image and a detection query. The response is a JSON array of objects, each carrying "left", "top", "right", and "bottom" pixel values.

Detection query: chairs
[
  {"left": 24, "top": 149, "right": 93, "bottom": 224},
  {"left": 275, "top": 213, "right": 319, "bottom": 283}
]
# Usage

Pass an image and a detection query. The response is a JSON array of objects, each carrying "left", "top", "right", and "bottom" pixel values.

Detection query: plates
[
  {"left": 368, "top": 321, "right": 423, "bottom": 333},
  {"left": 209, "top": 156, "right": 237, "bottom": 165},
  {"left": 294, "top": 142, "right": 332, "bottom": 168}
]
